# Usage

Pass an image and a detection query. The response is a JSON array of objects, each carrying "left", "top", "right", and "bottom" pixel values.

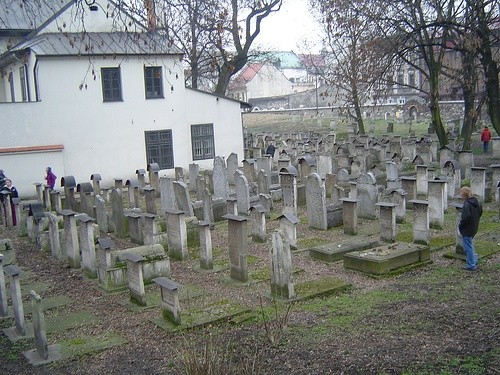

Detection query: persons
[
  {"left": 459, "top": 186, "right": 483, "bottom": 269},
  {"left": 0, "top": 179, "right": 18, "bottom": 226},
  {"left": 45, "top": 167, "right": 57, "bottom": 191},
  {"left": 0, "top": 169, "right": 7, "bottom": 203},
  {"left": 266, "top": 141, "right": 275, "bottom": 157},
  {"left": 481, "top": 126, "right": 491, "bottom": 153}
]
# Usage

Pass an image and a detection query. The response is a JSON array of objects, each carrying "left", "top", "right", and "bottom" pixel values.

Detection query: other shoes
[{"left": 463, "top": 265, "right": 477, "bottom": 271}]
